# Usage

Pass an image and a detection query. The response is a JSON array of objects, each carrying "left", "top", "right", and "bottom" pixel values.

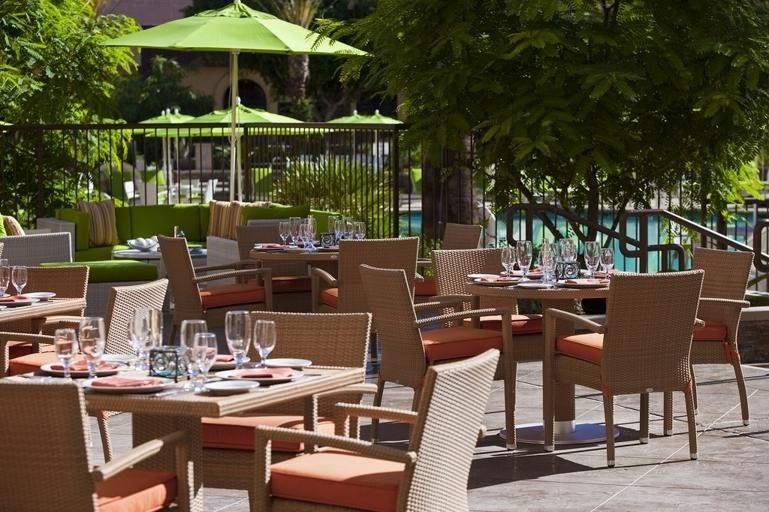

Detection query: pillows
[
  {"left": 3, "top": 215, "right": 26, "bottom": 236},
  {"left": 78, "top": 202, "right": 121, "bottom": 247},
  {"left": 208, "top": 202, "right": 245, "bottom": 240}
]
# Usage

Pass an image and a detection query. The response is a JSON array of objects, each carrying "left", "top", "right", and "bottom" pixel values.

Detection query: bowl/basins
[{"left": 127, "top": 238, "right": 159, "bottom": 251}]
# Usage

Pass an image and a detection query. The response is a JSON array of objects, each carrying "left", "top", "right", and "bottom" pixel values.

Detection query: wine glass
[
  {"left": 77, "top": 316, "right": 107, "bottom": 379},
  {"left": 328, "top": 215, "right": 366, "bottom": 244},
  {"left": 0, "top": 258, "right": 28, "bottom": 298},
  {"left": 278, "top": 216, "right": 318, "bottom": 251},
  {"left": 180, "top": 319, "right": 218, "bottom": 390},
  {"left": 53, "top": 327, "right": 78, "bottom": 380},
  {"left": 126, "top": 307, "right": 162, "bottom": 367},
  {"left": 500, "top": 238, "right": 614, "bottom": 282},
  {"left": 224, "top": 310, "right": 251, "bottom": 366},
  {"left": 253, "top": 320, "right": 277, "bottom": 366}
]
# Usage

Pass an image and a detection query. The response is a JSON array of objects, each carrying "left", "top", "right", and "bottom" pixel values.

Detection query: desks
[
  {"left": 2, "top": 350, "right": 364, "bottom": 512},
  {"left": 0, "top": 295, "right": 86, "bottom": 351},
  {"left": 248, "top": 247, "right": 339, "bottom": 313},
  {"left": 466, "top": 269, "right": 621, "bottom": 445}
]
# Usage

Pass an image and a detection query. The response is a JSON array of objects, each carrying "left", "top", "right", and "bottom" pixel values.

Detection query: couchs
[{"left": 0, "top": 200, "right": 342, "bottom": 318}]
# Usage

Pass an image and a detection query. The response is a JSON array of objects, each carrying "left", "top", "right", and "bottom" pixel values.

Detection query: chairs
[{"left": 0, "top": 224, "right": 755, "bottom": 511}]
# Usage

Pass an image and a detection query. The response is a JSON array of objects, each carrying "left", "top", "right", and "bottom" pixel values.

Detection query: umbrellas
[
  {"left": 97, "top": 0, "right": 375, "bottom": 203},
  {"left": 132, "top": 96, "right": 407, "bottom": 203}
]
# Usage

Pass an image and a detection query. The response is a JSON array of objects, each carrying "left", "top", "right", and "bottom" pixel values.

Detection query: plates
[
  {"left": 84, "top": 376, "right": 175, "bottom": 394},
  {"left": 264, "top": 357, "right": 313, "bottom": 369},
  {"left": 468, "top": 272, "right": 514, "bottom": 286},
  {"left": 211, "top": 355, "right": 252, "bottom": 370},
  {"left": 0, "top": 291, "right": 57, "bottom": 307},
  {"left": 319, "top": 246, "right": 339, "bottom": 251},
  {"left": 517, "top": 279, "right": 608, "bottom": 289},
  {"left": 253, "top": 243, "right": 280, "bottom": 251},
  {"left": 214, "top": 368, "right": 305, "bottom": 385},
  {"left": 204, "top": 380, "right": 261, "bottom": 394},
  {"left": 40, "top": 360, "right": 121, "bottom": 378}
]
